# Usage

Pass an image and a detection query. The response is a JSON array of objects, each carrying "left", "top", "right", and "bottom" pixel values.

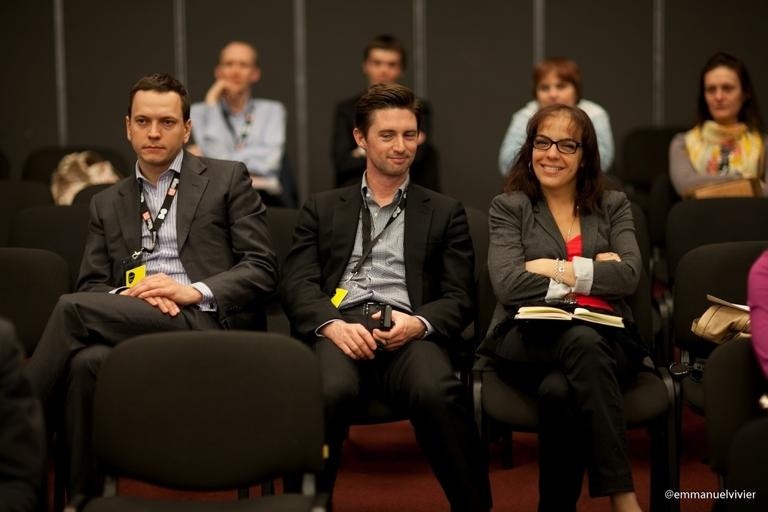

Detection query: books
[{"left": 514, "top": 304, "right": 626, "bottom": 329}]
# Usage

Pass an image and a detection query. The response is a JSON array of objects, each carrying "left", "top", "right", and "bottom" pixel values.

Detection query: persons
[
  {"left": 487, "top": 102, "right": 653, "bottom": 511},
  {"left": 332, "top": 34, "right": 446, "bottom": 190},
  {"left": 496, "top": 57, "right": 617, "bottom": 177},
  {"left": 723, "top": 249, "right": 768, "bottom": 510},
  {"left": 281, "top": 84, "right": 495, "bottom": 512},
  {"left": 669, "top": 50, "right": 766, "bottom": 201},
  {"left": 25, "top": 75, "right": 279, "bottom": 511},
  {"left": 187, "top": 40, "right": 289, "bottom": 208}
]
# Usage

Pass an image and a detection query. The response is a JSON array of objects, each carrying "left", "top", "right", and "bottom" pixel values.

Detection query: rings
[{"left": 384, "top": 337, "right": 392, "bottom": 346}]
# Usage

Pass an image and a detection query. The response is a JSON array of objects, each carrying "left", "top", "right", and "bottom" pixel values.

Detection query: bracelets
[
  {"left": 559, "top": 257, "right": 566, "bottom": 286},
  {"left": 552, "top": 255, "right": 558, "bottom": 285}
]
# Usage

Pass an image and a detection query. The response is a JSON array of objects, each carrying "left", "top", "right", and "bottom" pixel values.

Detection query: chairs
[{"left": 0, "top": 123, "right": 767, "bottom": 510}]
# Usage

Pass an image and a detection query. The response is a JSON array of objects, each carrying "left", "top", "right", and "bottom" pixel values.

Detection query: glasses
[{"left": 532, "top": 135, "right": 582, "bottom": 154}]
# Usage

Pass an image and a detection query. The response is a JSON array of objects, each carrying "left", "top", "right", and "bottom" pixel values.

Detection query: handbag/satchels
[{"left": 669, "top": 299, "right": 752, "bottom": 383}]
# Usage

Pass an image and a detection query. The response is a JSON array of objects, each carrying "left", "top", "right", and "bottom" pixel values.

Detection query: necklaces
[{"left": 545, "top": 199, "right": 582, "bottom": 245}]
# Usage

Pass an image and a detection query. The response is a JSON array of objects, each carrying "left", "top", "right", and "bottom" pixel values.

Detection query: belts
[{"left": 344, "top": 300, "right": 386, "bottom": 317}]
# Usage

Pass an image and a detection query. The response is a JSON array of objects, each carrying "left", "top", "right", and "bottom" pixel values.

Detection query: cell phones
[{"left": 380, "top": 305, "right": 392, "bottom": 331}]
